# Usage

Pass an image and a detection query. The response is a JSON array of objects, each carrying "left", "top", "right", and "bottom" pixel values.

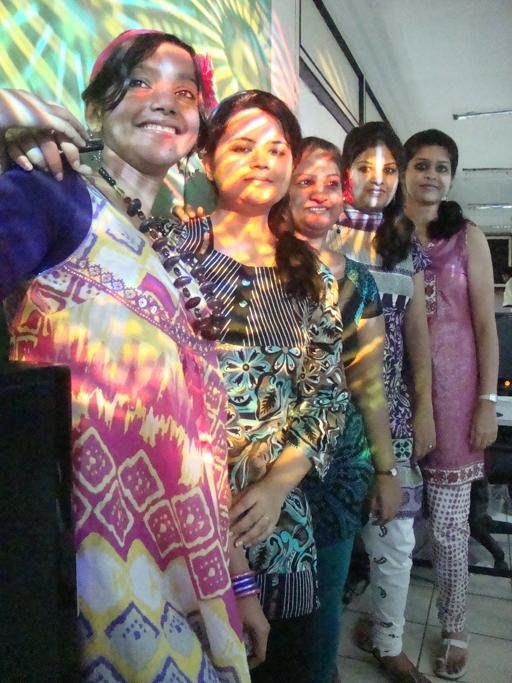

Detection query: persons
[
  {"left": 0, "top": 28, "right": 273, "bottom": 683},
  {"left": 396, "top": 128, "right": 500, "bottom": 681},
  {"left": 325, "top": 119, "right": 436, "bottom": 683},
  {"left": 500, "top": 265, "right": 512, "bottom": 308},
  {"left": 170, "top": 135, "right": 401, "bottom": 681},
  {"left": 3, "top": 88, "right": 350, "bottom": 683}
]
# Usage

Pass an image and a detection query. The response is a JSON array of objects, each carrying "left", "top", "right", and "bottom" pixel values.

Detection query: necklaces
[{"left": 100, "top": 167, "right": 228, "bottom": 341}]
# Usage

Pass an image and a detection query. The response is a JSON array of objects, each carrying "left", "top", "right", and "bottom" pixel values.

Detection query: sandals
[{"left": 353, "top": 622, "right": 432, "bottom": 683}]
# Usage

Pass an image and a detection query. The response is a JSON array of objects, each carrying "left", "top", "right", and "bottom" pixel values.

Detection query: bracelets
[
  {"left": 478, "top": 393, "right": 499, "bottom": 403},
  {"left": 230, "top": 571, "right": 262, "bottom": 600},
  {"left": 375, "top": 467, "right": 397, "bottom": 477}
]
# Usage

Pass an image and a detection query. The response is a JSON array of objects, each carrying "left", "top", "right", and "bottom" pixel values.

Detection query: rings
[{"left": 428, "top": 444, "right": 433, "bottom": 448}]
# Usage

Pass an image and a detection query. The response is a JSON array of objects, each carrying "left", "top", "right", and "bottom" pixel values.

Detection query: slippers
[{"left": 433, "top": 632, "right": 472, "bottom": 680}]
